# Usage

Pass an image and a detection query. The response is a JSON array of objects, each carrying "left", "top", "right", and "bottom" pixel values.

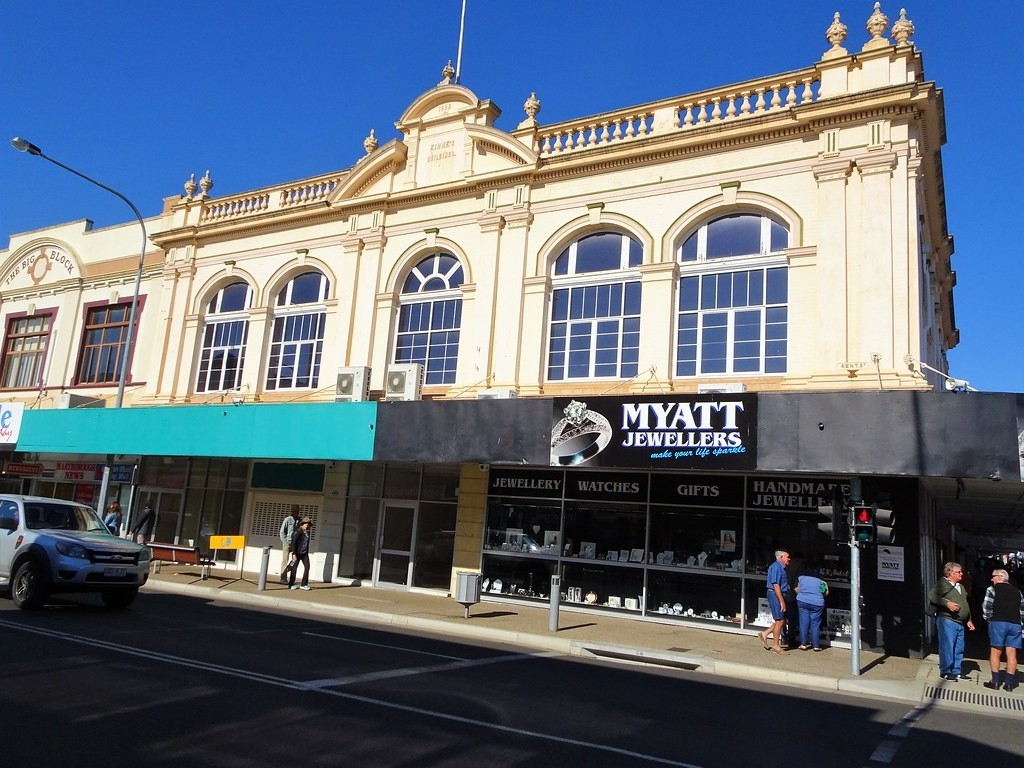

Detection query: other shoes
[
  {"left": 300, "top": 585, "right": 312, "bottom": 590},
  {"left": 280, "top": 579, "right": 289, "bottom": 584},
  {"left": 814, "top": 648, "right": 822, "bottom": 651},
  {"left": 758, "top": 633, "right": 770, "bottom": 650},
  {"left": 770, "top": 647, "right": 790, "bottom": 656},
  {"left": 984, "top": 681, "right": 999, "bottom": 690},
  {"left": 1003, "top": 684, "right": 1012, "bottom": 691},
  {"left": 288, "top": 585, "right": 298, "bottom": 589},
  {"left": 799, "top": 645, "right": 807, "bottom": 650}
]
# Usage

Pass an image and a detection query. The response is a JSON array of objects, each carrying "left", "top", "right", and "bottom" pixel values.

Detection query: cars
[{"left": 416, "top": 530, "right": 541, "bottom": 568}]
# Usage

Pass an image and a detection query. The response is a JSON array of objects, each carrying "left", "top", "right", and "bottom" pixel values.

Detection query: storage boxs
[
  {"left": 575, "top": 588, "right": 582, "bottom": 602},
  {"left": 568, "top": 587, "right": 575, "bottom": 601},
  {"left": 608, "top": 597, "right": 621, "bottom": 607},
  {"left": 625, "top": 598, "right": 638, "bottom": 609}
]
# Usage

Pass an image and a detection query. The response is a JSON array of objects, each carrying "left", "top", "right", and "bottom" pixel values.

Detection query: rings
[{"left": 551, "top": 400, "right": 612, "bottom": 466}]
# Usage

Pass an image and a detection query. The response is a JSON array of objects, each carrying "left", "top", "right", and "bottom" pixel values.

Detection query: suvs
[{"left": 0, "top": 494, "right": 150, "bottom": 608}]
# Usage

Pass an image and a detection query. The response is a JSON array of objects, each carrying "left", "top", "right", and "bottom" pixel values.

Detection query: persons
[
  {"left": 928, "top": 562, "right": 975, "bottom": 682},
  {"left": 287, "top": 517, "right": 313, "bottom": 590},
  {"left": 279, "top": 506, "right": 302, "bottom": 584},
  {"left": 757, "top": 548, "right": 791, "bottom": 655},
  {"left": 724, "top": 534, "right": 735, "bottom": 544},
  {"left": 796, "top": 561, "right": 829, "bottom": 651},
  {"left": 105, "top": 501, "right": 122, "bottom": 535},
  {"left": 130, "top": 500, "right": 156, "bottom": 545},
  {"left": 982, "top": 569, "right": 1024, "bottom": 693}
]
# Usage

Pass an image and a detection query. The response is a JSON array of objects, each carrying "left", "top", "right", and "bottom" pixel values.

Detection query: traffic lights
[
  {"left": 851, "top": 505, "right": 877, "bottom": 543},
  {"left": 875, "top": 505, "right": 896, "bottom": 545},
  {"left": 813, "top": 488, "right": 843, "bottom": 541}
]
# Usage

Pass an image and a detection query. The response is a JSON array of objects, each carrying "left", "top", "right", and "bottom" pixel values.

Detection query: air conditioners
[
  {"left": 334, "top": 367, "right": 372, "bottom": 402},
  {"left": 698, "top": 383, "right": 748, "bottom": 394},
  {"left": 476, "top": 389, "right": 518, "bottom": 399},
  {"left": 386, "top": 363, "right": 425, "bottom": 401},
  {"left": 57, "top": 393, "right": 107, "bottom": 408}
]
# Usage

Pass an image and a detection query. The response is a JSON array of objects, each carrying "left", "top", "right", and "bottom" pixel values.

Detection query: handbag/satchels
[{"left": 925, "top": 583, "right": 956, "bottom": 617}]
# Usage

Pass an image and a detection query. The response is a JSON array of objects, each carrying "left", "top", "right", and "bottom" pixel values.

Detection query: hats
[{"left": 300, "top": 518, "right": 313, "bottom": 528}]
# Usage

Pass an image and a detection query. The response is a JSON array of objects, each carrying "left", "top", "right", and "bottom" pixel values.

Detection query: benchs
[{"left": 143, "top": 541, "right": 216, "bottom": 581}]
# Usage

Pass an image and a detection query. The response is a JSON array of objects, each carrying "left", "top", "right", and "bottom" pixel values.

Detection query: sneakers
[
  {"left": 955, "top": 673, "right": 971, "bottom": 681},
  {"left": 939, "top": 673, "right": 957, "bottom": 681}
]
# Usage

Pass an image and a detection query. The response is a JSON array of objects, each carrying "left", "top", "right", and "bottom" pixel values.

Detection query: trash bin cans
[{"left": 455, "top": 571, "right": 483, "bottom": 607}]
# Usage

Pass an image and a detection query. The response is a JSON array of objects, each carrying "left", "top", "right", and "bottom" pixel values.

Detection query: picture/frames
[
  {"left": 506, "top": 528, "right": 523, "bottom": 551},
  {"left": 545, "top": 531, "right": 560, "bottom": 555},
  {"left": 580, "top": 542, "right": 596, "bottom": 559}
]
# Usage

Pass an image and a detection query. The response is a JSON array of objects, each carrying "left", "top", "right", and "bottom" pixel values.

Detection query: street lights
[{"left": 9, "top": 137, "right": 146, "bottom": 519}]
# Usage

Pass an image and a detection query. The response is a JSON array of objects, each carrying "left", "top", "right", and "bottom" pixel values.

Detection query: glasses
[{"left": 991, "top": 575, "right": 1002, "bottom": 579}]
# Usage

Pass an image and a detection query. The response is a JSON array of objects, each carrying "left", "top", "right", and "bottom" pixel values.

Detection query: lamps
[
  {"left": 945, "top": 378, "right": 969, "bottom": 391},
  {"left": 231, "top": 396, "right": 245, "bottom": 405}
]
